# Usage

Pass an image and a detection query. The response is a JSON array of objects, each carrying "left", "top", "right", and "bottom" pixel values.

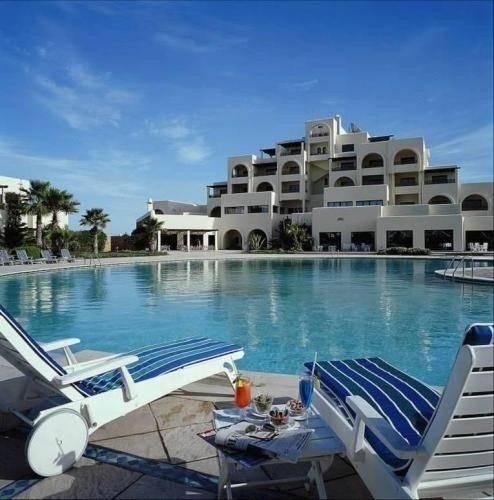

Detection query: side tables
[{"left": 211, "top": 402, "right": 348, "bottom": 500}]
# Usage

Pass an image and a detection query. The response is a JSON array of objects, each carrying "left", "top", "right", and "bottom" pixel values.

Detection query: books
[{"left": 195, "top": 421, "right": 277, "bottom": 469}]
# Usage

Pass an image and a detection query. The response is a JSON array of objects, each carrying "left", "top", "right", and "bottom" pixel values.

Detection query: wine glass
[
  {"left": 235, "top": 375, "right": 251, "bottom": 422},
  {"left": 299, "top": 375, "right": 312, "bottom": 429}
]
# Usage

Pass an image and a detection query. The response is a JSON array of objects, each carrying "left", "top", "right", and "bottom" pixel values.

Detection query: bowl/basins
[{"left": 253, "top": 392, "right": 305, "bottom": 432}]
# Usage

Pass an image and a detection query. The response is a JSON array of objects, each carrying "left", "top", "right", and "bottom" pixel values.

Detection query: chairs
[
  {"left": 468, "top": 242, "right": 488, "bottom": 252},
  {"left": 0, "top": 249, "right": 75, "bottom": 267},
  {"left": 302, "top": 321, "right": 494, "bottom": 500},
  {"left": 0, "top": 301, "right": 246, "bottom": 478}
]
